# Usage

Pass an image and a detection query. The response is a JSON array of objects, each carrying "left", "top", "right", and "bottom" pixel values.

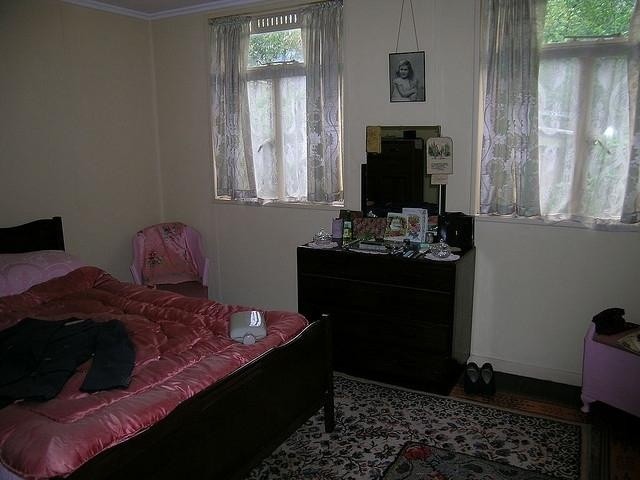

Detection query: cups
[{"left": 425, "top": 232, "right": 434, "bottom": 244}]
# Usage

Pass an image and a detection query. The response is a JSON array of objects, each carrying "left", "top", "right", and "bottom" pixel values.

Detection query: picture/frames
[{"left": 389, "top": 51, "right": 425, "bottom": 102}]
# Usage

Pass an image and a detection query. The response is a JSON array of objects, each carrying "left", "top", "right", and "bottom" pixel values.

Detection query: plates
[{"left": 425, "top": 254, "right": 461, "bottom": 262}]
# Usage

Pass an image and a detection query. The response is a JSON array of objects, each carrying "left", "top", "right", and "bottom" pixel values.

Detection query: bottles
[
  {"left": 332, "top": 217, "right": 342, "bottom": 239},
  {"left": 343, "top": 209, "right": 352, "bottom": 233},
  {"left": 343, "top": 229, "right": 350, "bottom": 246}
]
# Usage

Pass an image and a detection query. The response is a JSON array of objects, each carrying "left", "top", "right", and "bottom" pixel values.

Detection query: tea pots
[
  {"left": 432, "top": 240, "right": 451, "bottom": 259},
  {"left": 312, "top": 226, "right": 333, "bottom": 245}
]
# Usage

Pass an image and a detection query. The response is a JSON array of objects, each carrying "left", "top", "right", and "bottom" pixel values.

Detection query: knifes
[{"left": 402, "top": 248, "right": 429, "bottom": 259}]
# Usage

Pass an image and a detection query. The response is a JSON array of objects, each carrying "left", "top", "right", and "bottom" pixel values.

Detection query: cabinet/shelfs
[{"left": 297, "top": 210, "right": 476, "bottom": 395}]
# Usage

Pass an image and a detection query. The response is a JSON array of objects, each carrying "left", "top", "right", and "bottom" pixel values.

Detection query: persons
[{"left": 392, "top": 59, "right": 418, "bottom": 102}]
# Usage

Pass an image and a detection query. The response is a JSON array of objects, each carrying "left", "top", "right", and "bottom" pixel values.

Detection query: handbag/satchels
[{"left": 437, "top": 215, "right": 474, "bottom": 248}]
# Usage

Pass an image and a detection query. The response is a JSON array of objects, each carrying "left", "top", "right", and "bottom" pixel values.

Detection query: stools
[{"left": 581, "top": 308, "right": 640, "bottom": 417}]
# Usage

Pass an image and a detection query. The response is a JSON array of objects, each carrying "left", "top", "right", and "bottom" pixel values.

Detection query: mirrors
[{"left": 367, "top": 127, "right": 439, "bottom": 218}]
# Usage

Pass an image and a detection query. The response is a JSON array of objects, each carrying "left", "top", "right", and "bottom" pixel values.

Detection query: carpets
[{"left": 240, "top": 371, "right": 640, "bottom": 479}]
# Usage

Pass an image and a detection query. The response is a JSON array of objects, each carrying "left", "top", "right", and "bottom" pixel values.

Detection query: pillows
[{"left": 0, "top": 250, "right": 89, "bottom": 297}]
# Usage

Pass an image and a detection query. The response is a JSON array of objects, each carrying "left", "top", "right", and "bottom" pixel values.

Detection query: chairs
[{"left": 129, "top": 223, "right": 209, "bottom": 298}]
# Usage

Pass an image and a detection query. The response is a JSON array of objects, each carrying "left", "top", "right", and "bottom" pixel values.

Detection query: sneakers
[
  {"left": 481, "top": 363, "right": 496, "bottom": 394},
  {"left": 464, "top": 362, "right": 480, "bottom": 393}
]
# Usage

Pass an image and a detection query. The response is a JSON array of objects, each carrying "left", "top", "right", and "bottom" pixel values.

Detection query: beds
[{"left": 0, "top": 217, "right": 335, "bottom": 479}]
